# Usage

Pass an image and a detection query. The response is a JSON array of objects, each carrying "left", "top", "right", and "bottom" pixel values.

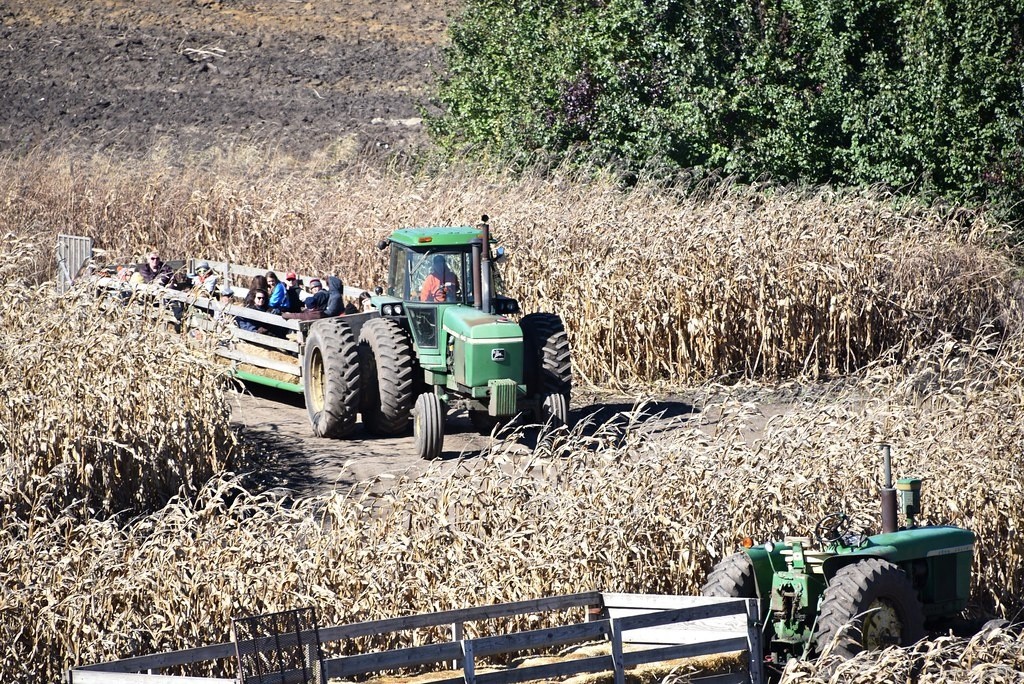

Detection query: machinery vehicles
[
  {"left": 65, "top": 446, "right": 977, "bottom": 684},
  {"left": 92, "top": 214, "right": 573, "bottom": 460}
]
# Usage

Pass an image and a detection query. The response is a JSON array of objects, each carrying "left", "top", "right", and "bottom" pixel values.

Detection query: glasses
[
  {"left": 151, "top": 257, "right": 160, "bottom": 261},
  {"left": 256, "top": 296, "right": 265, "bottom": 300},
  {"left": 198, "top": 270, "right": 206, "bottom": 273}
]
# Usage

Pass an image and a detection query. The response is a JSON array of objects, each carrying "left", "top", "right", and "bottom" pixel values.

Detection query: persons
[
  {"left": 419, "top": 255, "right": 460, "bottom": 302},
  {"left": 96, "top": 252, "right": 235, "bottom": 335},
  {"left": 237, "top": 270, "right": 373, "bottom": 358}
]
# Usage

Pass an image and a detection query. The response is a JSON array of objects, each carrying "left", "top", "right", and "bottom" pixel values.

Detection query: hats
[
  {"left": 434, "top": 256, "right": 445, "bottom": 263},
  {"left": 285, "top": 273, "right": 296, "bottom": 280},
  {"left": 195, "top": 261, "right": 211, "bottom": 271},
  {"left": 310, "top": 282, "right": 322, "bottom": 289},
  {"left": 304, "top": 297, "right": 316, "bottom": 306}
]
